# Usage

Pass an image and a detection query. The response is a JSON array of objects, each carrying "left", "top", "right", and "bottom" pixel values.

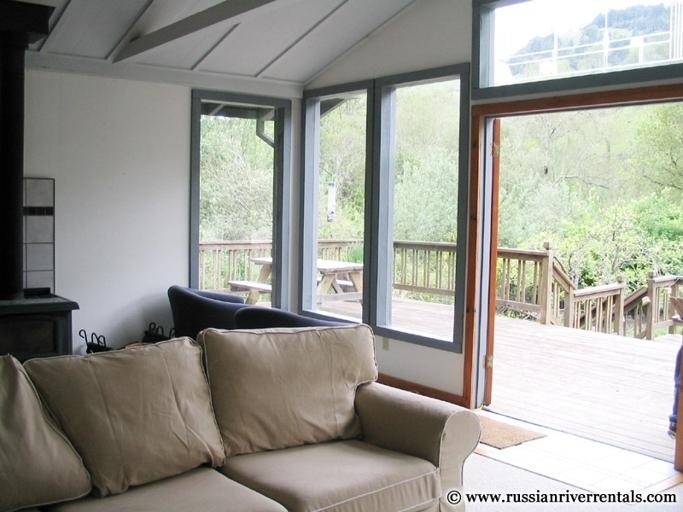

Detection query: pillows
[
  {"left": 196, "top": 324, "right": 378, "bottom": 458},
  {"left": 0, "top": 353, "right": 93, "bottom": 512},
  {"left": 22, "top": 336, "right": 226, "bottom": 498}
]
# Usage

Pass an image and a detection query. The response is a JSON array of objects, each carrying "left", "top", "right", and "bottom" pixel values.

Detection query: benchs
[
  {"left": 228, "top": 281, "right": 272, "bottom": 304},
  {"left": 317, "top": 277, "right": 353, "bottom": 286}
]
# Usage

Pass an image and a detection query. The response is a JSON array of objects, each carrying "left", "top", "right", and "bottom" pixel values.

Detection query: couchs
[{"left": 0, "top": 323, "right": 482, "bottom": 512}]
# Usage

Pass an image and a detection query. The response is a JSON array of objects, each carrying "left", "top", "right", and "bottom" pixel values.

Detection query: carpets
[{"left": 477, "top": 415, "right": 547, "bottom": 449}]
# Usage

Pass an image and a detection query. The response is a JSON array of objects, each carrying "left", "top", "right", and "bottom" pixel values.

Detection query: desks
[{"left": 245, "top": 257, "right": 364, "bottom": 304}]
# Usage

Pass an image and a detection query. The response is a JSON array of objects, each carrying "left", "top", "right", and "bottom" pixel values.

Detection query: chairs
[
  {"left": 232, "top": 307, "right": 349, "bottom": 329},
  {"left": 168, "top": 285, "right": 249, "bottom": 340}
]
